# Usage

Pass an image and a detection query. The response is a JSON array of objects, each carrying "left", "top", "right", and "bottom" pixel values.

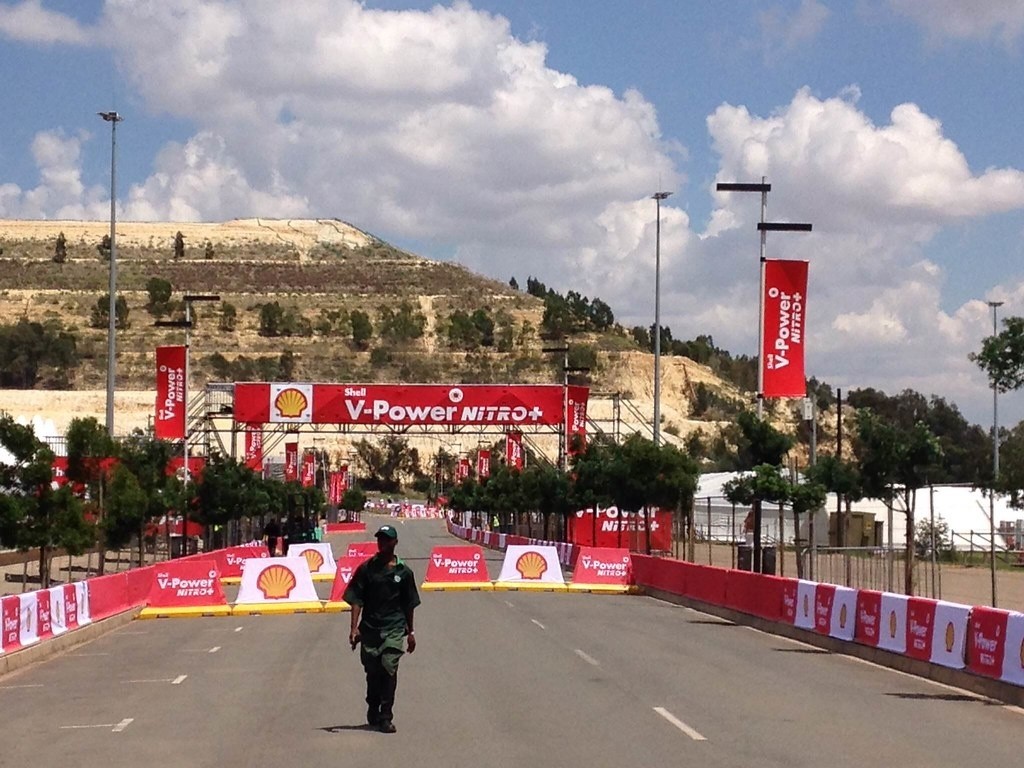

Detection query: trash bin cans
[{"left": 736, "top": 543, "right": 776, "bottom": 575}]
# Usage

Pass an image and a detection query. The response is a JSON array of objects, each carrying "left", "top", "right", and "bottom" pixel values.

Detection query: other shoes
[
  {"left": 368, "top": 702, "right": 380, "bottom": 725},
  {"left": 374, "top": 712, "right": 396, "bottom": 733}
]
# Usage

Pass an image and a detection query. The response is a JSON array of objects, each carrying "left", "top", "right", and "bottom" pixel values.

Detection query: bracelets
[{"left": 410, "top": 628, "right": 416, "bottom": 635}]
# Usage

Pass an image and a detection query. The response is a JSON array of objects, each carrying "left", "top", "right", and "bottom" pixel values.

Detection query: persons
[
  {"left": 282, "top": 523, "right": 289, "bottom": 532},
  {"left": 493, "top": 516, "right": 500, "bottom": 528},
  {"left": 304, "top": 518, "right": 315, "bottom": 529},
  {"left": 470, "top": 514, "right": 482, "bottom": 530},
  {"left": 342, "top": 525, "right": 421, "bottom": 733},
  {"left": 264, "top": 519, "right": 280, "bottom": 556}
]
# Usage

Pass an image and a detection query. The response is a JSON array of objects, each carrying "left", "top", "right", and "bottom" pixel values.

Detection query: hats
[{"left": 374, "top": 525, "right": 397, "bottom": 541}]
